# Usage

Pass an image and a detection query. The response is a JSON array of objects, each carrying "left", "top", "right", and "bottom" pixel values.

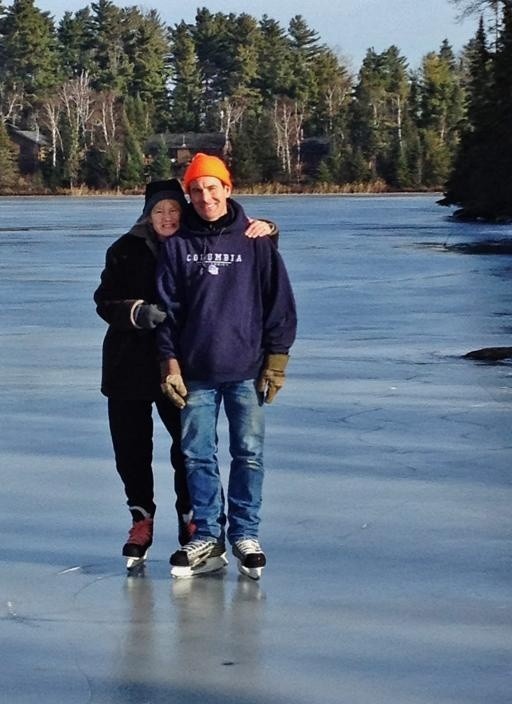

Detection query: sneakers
[
  {"left": 170, "top": 540, "right": 227, "bottom": 567},
  {"left": 232, "top": 538, "right": 266, "bottom": 567}
]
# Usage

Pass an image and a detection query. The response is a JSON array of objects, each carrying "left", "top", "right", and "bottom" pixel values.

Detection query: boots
[
  {"left": 174, "top": 502, "right": 197, "bottom": 543},
  {"left": 120, "top": 504, "right": 155, "bottom": 555}
]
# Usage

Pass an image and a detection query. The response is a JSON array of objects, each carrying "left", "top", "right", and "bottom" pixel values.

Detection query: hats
[
  {"left": 146, "top": 179, "right": 188, "bottom": 214},
  {"left": 182, "top": 153, "right": 232, "bottom": 191}
]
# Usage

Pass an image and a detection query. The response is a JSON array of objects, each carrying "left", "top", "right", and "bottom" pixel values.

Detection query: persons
[
  {"left": 150, "top": 152, "right": 297, "bottom": 580},
  {"left": 94, "top": 178, "right": 279, "bottom": 574}
]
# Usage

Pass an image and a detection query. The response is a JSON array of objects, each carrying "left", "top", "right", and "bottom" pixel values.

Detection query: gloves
[
  {"left": 135, "top": 301, "right": 166, "bottom": 329},
  {"left": 159, "top": 359, "right": 187, "bottom": 410},
  {"left": 259, "top": 354, "right": 291, "bottom": 402}
]
